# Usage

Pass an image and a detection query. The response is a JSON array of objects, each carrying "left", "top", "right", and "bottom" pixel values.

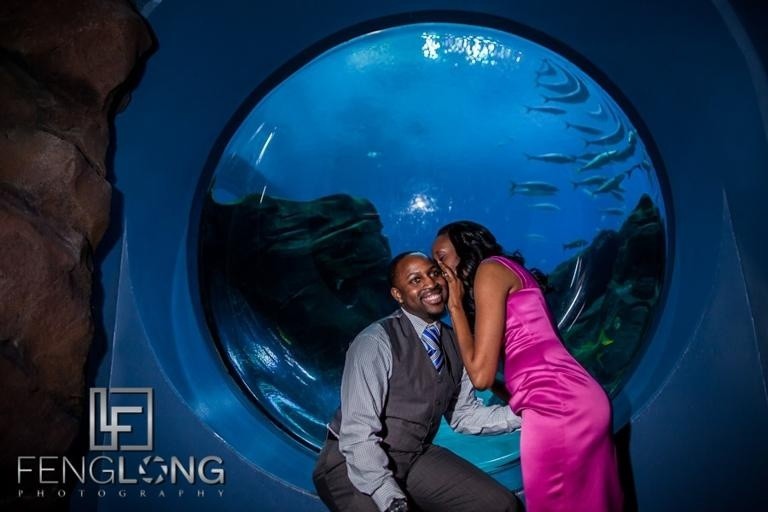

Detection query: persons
[
  {"left": 313, "top": 252, "right": 524, "bottom": 512},
  {"left": 432, "top": 221, "right": 624, "bottom": 512}
]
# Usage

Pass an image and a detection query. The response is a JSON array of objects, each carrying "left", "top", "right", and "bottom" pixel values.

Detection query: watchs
[{"left": 385, "top": 498, "right": 408, "bottom": 512}]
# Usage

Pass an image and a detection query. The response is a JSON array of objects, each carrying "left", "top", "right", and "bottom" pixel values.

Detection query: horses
[{"left": 570, "top": 328, "right": 615, "bottom": 361}]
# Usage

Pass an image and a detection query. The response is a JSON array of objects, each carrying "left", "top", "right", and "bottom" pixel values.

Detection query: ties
[{"left": 421, "top": 327, "right": 444, "bottom": 376}]
